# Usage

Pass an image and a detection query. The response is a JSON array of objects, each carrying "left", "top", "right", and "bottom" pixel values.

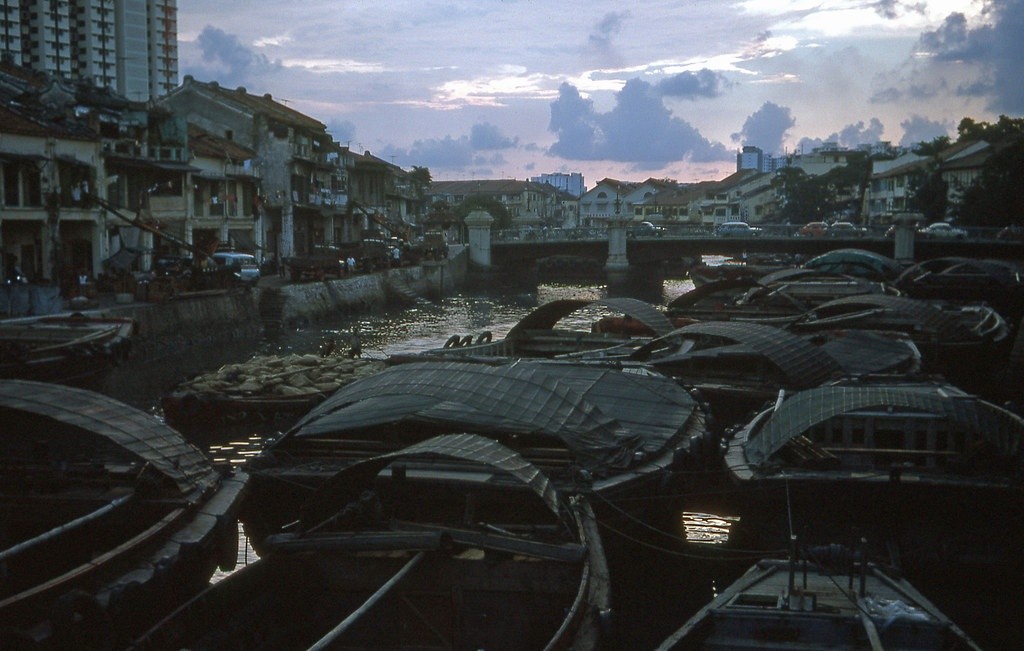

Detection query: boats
[
  {"left": 128, "top": 433, "right": 611, "bottom": 651},
  {"left": 159, "top": 351, "right": 402, "bottom": 436},
  {"left": 426, "top": 251, "right": 1024, "bottom": 415},
  {"left": 0, "top": 379, "right": 251, "bottom": 651},
  {"left": 654, "top": 535, "right": 982, "bottom": 651},
  {"left": 247, "top": 354, "right": 715, "bottom": 527},
  {"left": 716, "top": 370, "right": 1024, "bottom": 581},
  {"left": 0, "top": 315, "right": 137, "bottom": 379}
]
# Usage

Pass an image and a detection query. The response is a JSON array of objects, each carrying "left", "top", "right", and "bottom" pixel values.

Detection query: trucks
[
  {"left": 421, "top": 230, "right": 449, "bottom": 258},
  {"left": 626, "top": 222, "right": 667, "bottom": 237}
]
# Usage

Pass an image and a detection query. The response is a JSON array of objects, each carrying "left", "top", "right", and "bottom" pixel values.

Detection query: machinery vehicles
[
  {"left": 83, "top": 192, "right": 248, "bottom": 267},
  {"left": 348, "top": 198, "right": 412, "bottom": 249}
]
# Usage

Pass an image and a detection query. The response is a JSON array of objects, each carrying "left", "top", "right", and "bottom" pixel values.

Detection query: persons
[
  {"left": 79, "top": 272, "right": 88, "bottom": 297},
  {"left": 390, "top": 246, "right": 401, "bottom": 267},
  {"left": 337, "top": 256, "right": 364, "bottom": 273},
  {"left": 175, "top": 257, "right": 184, "bottom": 275},
  {"left": 151, "top": 267, "right": 158, "bottom": 278},
  {"left": 351, "top": 327, "right": 362, "bottom": 359},
  {"left": 278, "top": 253, "right": 285, "bottom": 279},
  {"left": 543, "top": 224, "right": 548, "bottom": 234},
  {"left": 320, "top": 339, "right": 337, "bottom": 358},
  {"left": 527, "top": 224, "right": 532, "bottom": 233}
]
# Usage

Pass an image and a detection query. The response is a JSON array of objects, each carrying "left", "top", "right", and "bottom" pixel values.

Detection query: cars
[
  {"left": 718, "top": 222, "right": 763, "bottom": 236},
  {"left": 884, "top": 224, "right": 936, "bottom": 239},
  {"left": 923, "top": 223, "right": 968, "bottom": 238},
  {"left": 997, "top": 224, "right": 1024, "bottom": 239},
  {"left": 800, "top": 222, "right": 828, "bottom": 236},
  {"left": 824, "top": 222, "right": 867, "bottom": 237},
  {"left": 183, "top": 253, "right": 261, "bottom": 286}
]
